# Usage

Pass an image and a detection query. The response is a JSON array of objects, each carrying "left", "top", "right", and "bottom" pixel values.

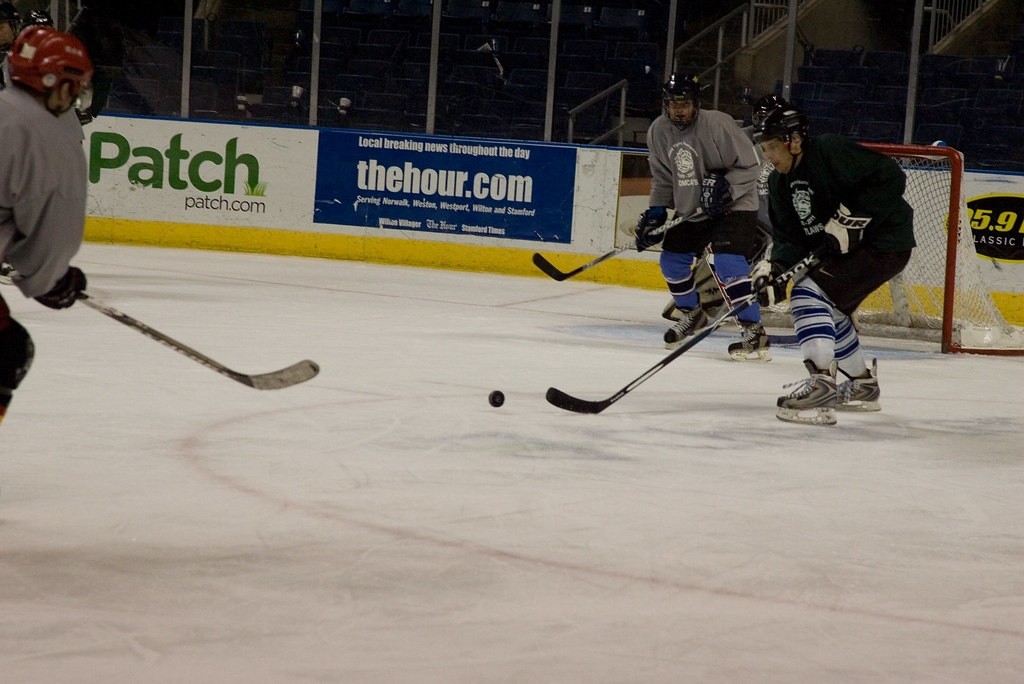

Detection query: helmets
[
  {"left": 4, "top": 26, "right": 91, "bottom": 113},
  {"left": 663, "top": 73, "right": 702, "bottom": 127},
  {"left": 751, "top": 93, "right": 803, "bottom": 142}
]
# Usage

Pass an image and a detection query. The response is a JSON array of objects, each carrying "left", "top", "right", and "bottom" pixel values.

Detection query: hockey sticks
[
  {"left": 545, "top": 251, "right": 817, "bottom": 415},
  {"left": 531, "top": 206, "right": 706, "bottom": 282},
  {"left": 3, "top": 265, "right": 320, "bottom": 391}
]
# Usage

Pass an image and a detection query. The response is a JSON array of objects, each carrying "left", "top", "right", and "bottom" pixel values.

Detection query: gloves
[
  {"left": 33, "top": 266, "right": 87, "bottom": 310},
  {"left": 635, "top": 210, "right": 666, "bottom": 252},
  {"left": 700, "top": 173, "right": 731, "bottom": 216},
  {"left": 750, "top": 263, "right": 792, "bottom": 307},
  {"left": 811, "top": 219, "right": 863, "bottom": 262}
]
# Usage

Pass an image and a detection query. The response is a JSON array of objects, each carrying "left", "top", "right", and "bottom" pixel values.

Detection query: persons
[
  {"left": 753, "top": 94, "right": 917, "bottom": 426},
  {"left": 635, "top": 73, "right": 786, "bottom": 363},
  {"left": 0, "top": 24, "right": 96, "bottom": 415}
]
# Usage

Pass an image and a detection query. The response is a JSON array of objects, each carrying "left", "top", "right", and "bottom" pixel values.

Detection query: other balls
[{"left": 489, "top": 390, "right": 505, "bottom": 407}]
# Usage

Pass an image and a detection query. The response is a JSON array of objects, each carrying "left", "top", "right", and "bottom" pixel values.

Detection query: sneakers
[
  {"left": 777, "top": 359, "right": 836, "bottom": 426},
  {"left": 664, "top": 291, "right": 708, "bottom": 350},
  {"left": 837, "top": 358, "right": 882, "bottom": 411},
  {"left": 728, "top": 327, "right": 772, "bottom": 362}
]
